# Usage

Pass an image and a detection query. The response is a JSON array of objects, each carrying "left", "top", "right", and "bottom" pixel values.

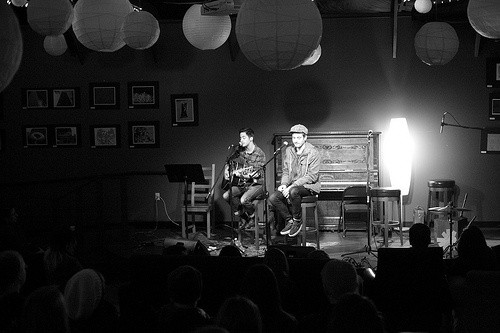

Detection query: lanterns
[
  {"left": 26, "top": 0, "right": 73, "bottom": 37},
  {"left": 415, "top": 21, "right": 459, "bottom": 67},
  {"left": 72, "top": 0, "right": 133, "bottom": 52},
  {"left": 235, "top": 0, "right": 322, "bottom": 70},
  {"left": 414, "top": 0, "right": 433, "bottom": 13},
  {"left": 182, "top": 4, "right": 231, "bottom": 51},
  {"left": 301, "top": 43, "right": 321, "bottom": 65},
  {"left": 0, "top": 3, "right": 23, "bottom": 94},
  {"left": 121, "top": 10, "right": 160, "bottom": 50},
  {"left": 44, "top": 33, "right": 68, "bottom": 56},
  {"left": 467, "top": 1, "right": 500, "bottom": 38}
]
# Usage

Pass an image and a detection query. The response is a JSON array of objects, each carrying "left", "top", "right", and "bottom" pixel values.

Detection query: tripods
[
  {"left": 443, "top": 213, "right": 459, "bottom": 259},
  {"left": 341, "top": 138, "right": 379, "bottom": 258}
]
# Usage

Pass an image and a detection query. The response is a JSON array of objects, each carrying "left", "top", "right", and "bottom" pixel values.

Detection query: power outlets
[{"left": 155, "top": 193, "right": 160, "bottom": 200}]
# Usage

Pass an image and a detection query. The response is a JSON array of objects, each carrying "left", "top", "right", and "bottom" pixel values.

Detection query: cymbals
[{"left": 428, "top": 207, "right": 473, "bottom": 212}]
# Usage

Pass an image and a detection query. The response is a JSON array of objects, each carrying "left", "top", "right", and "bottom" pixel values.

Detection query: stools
[
  {"left": 427, "top": 180, "right": 456, "bottom": 228},
  {"left": 368, "top": 188, "right": 403, "bottom": 248},
  {"left": 285, "top": 195, "right": 319, "bottom": 250},
  {"left": 239, "top": 191, "right": 269, "bottom": 246}
]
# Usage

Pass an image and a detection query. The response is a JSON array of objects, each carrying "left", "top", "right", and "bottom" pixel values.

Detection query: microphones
[
  {"left": 277, "top": 141, "right": 289, "bottom": 151},
  {"left": 440, "top": 114, "right": 445, "bottom": 133},
  {"left": 367, "top": 130, "right": 373, "bottom": 138},
  {"left": 232, "top": 142, "right": 242, "bottom": 147}
]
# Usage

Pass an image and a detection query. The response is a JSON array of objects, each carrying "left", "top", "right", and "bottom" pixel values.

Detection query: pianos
[{"left": 272, "top": 130, "right": 380, "bottom": 231}]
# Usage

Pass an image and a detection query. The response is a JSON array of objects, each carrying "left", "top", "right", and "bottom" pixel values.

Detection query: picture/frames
[
  {"left": 481, "top": 127, "right": 500, "bottom": 154},
  {"left": 488, "top": 93, "right": 500, "bottom": 121},
  {"left": 486, "top": 58, "right": 500, "bottom": 88},
  {"left": 171, "top": 94, "right": 199, "bottom": 127},
  {"left": 127, "top": 80, "right": 160, "bottom": 109},
  {"left": 21, "top": 123, "right": 81, "bottom": 148},
  {"left": 20, "top": 87, "right": 81, "bottom": 110},
  {"left": 89, "top": 82, "right": 121, "bottom": 110},
  {"left": 90, "top": 123, "right": 121, "bottom": 150},
  {"left": 128, "top": 120, "right": 160, "bottom": 149}
]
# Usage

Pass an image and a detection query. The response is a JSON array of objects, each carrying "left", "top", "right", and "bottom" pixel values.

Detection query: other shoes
[
  {"left": 237, "top": 215, "right": 248, "bottom": 230},
  {"left": 246, "top": 217, "right": 259, "bottom": 229}
]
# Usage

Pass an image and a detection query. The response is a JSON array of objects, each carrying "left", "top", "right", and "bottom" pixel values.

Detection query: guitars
[{"left": 221, "top": 161, "right": 261, "bottom": 190}]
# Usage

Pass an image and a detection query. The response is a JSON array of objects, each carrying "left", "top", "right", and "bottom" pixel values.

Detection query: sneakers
[
  {"left": 280, "top": 222, "right": 293, "bottom": 235},
  {"left": 289, "top": 220, "right": 302, "bottom": 236}
]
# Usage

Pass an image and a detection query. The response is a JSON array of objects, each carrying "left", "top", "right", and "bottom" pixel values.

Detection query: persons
[
  {"left": 0, "top": 226, "right": 500, "bottom": 333},
  {"left": 409, "top": 223, "right": 432, "bottom": 250},
  {"left": 269, "top": 124, "right": 321, "bottom": 237},
  {"left": 223, "top": 128, "right": 267, "bottom": 218}
]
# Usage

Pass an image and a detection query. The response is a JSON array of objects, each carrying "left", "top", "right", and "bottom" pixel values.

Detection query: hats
[
  {"left": 289, "top": 124, "right": 308, "bottom": 134},
  {"left": 64, "top": 269, "right": 104, "bottom": 319}
]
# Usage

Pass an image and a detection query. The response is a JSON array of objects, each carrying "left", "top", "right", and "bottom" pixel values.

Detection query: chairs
[
  {"left": 181, "top": 164, "right": 216, "bottom": 239},
  {"left": 341, "top": 185, "right": 371, "bottom": 238},
  {"left": 376, "top": 248, "right": 443, "bottom": 313}
]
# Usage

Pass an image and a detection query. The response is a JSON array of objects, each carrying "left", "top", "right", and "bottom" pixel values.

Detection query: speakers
[
  {"left": 434, "top": 216, "right": 468, "bottom": 241},
  {"left": 269, "top": 244, "right": 315, "bottom": 270},
  {"left": 374, "top": 247, "right": 443, "bottom": 281},
  {"left": 165, "top": 239, "right": 211, "bottom": 258}
]
